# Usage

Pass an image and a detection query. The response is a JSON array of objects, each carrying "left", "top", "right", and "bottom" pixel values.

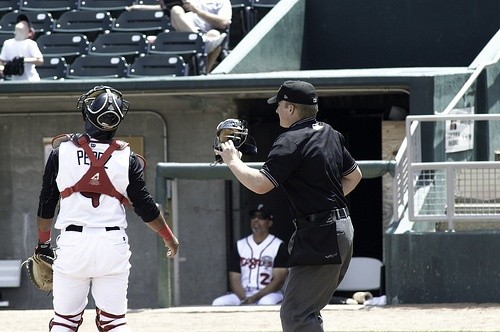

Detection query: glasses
[{"left": 250, "top": 212, "right": 268, "bottom": 220}]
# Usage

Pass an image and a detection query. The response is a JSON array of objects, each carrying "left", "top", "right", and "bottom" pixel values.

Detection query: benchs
[{"left": 0, "top": 260, "right": 22, "bottom": 307}]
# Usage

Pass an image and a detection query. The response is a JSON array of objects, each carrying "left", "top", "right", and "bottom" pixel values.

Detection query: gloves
[{"left": 35, "top": 240, "right": 55, "bottom": 264}]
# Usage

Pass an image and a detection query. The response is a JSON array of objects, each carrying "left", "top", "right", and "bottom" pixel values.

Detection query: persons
[
  {"left": 211, "top": 204, "right": 288, "bottom": 306},
  {"left": 214, "top": 80, "right": 362, "bottom": 332},
  {"left": 172, "top": 0, "right": 232, "bottom": 74},
  {"left": 0, "top": 14, "right": 44, "bottom": 82},
  {"left": 27, "top": 84, "right": 180, "bottom": 332}
]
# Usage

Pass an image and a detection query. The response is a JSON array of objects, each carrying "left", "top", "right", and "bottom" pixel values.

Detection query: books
[{"left": 158, "top": 0, "right": 191, "bottom": 16}]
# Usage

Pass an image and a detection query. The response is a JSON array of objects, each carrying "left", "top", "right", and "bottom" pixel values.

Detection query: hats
[
  {"left": 248, "top": 203, "right": 275, "bottom": 219},
  {"left": 267, "top": 80, "right": 317, "bottom": 106},
  {"left": 17, "top": 13, "right": 34, "bottom": 38}
]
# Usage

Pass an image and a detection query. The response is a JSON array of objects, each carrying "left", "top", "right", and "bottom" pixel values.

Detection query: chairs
[
  {"left": 436, "top": 169, "right": 500, "bottom": 231},
  {"left": 0, "top": 0, "right": 279, "bottom": 81},
  {"left": 335, "top": 257, "right": 385, "bottom": 297}
]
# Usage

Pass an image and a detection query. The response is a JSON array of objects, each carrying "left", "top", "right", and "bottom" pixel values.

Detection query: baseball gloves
[{"left": 20, "top": 246, "right": 55, "bottom": 298}]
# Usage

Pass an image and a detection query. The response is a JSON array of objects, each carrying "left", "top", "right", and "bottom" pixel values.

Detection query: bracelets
[
  {"left": 157, "top": 224, "right": 174, "bottom": 241},
  {"left": 37, "top": 229, "right": 51, "bottom": 242}
]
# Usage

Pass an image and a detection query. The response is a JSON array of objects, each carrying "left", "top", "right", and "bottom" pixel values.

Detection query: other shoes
[{"left": 204, "top": 33, "right": 227, "bottom": 56}]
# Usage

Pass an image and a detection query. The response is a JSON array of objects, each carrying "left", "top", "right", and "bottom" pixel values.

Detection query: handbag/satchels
[{"left": 287, "top": 220, "right": 342, "bottom": 265}]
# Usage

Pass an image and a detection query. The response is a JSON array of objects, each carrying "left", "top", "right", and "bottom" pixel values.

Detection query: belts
[
  {"left": 294, "top": 207, "right": 349, "bottom": 228},
  {"left": 66, "top": 224, "right": 120, "bottom": 233}
]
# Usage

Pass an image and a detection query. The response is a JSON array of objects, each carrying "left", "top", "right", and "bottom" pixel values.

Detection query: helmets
[{"left": 85, "top": 92, "right": 122, "bottom": 141}]
